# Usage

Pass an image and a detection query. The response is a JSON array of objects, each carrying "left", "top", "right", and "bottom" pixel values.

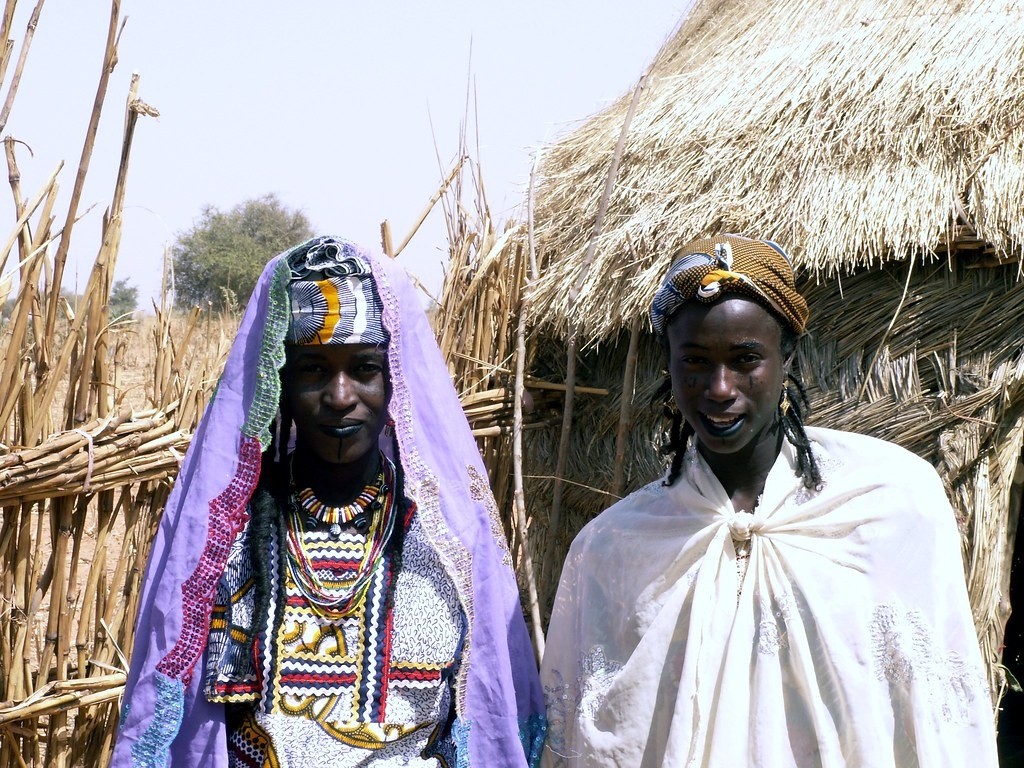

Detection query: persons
[
  {"left": 111, "top": 235, "right": 550, "bottom": 768},
  {"left": 538, "top": 234, "right": 999, "bottom": 768}
]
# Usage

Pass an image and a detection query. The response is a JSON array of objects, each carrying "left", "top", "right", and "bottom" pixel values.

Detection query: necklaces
[
  {"left": 290, "top": 463, "right": 389, "bottom": 536},
  {"left": 285, "top": 450, "right": 396, "bottom": 619}
]
